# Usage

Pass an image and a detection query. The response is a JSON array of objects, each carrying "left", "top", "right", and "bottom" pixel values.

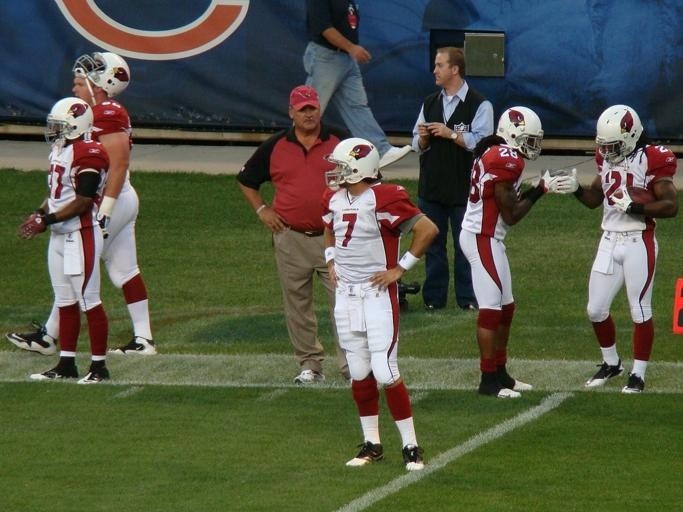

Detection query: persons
[
  {"left": 13, "top": 96, "right": 113, "bottom": 386},
  {"left": 236, "top": 85, "right": 352, "bottom": 383},
  {"left": 555, "top": 102, "right": 679, "bottom": 396},
  {"left": 456, "top": 107, "right": 555, "bottom": 397},
  {"left": 410, "top": 48, "right": 493, "bottom": 315},
  {"left": 319, "top": 135, "right": 441, "bottom": 471},
  {"left": 300, "top": 1, "right": 409, "bottom": 171},
  {"left": 4, "top": 50, "right": 157, "bottom": 358}
]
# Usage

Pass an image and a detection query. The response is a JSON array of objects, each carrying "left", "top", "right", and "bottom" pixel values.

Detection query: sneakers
[
  {"left": 295, "top": 371, "right": 326, "bottom": 384},
  {"left": 344, "top": 441, "right": 382, "bottom": 467},
  {"left": 377, "top": 145, "right": 412, "bottom": 170},
  {"left": 622, "top": 369, "right": 646, "bottom": 395},
  {"left": 109, "top": 338, "right": 157, "bottom": 357},
  {"left": 500, "top": 371, "right": 533, "bottom": 392},
  {"left": 30, "top": 357, "right": 77, "bottom": 381},
  {"left": 401, "top": 444, "right": 426, "bottom": 472},
  {"left": 6, "top": 330, "right": 57, "bottom": 355},
  {"left": 584, "top": 359, "right": 625, "bottom": 387},
  {"left": 77, "top": 360, "right": 110, "bottom": 384},
  {"left": 478, "top": 382, "right": 522, "bottom": 400}
]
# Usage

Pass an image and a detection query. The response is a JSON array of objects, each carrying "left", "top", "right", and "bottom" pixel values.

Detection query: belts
[{"left": 282, "top": 223, "right": 326, "bottom": 237}]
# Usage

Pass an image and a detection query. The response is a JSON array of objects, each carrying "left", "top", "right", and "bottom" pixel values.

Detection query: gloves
[
  {"left": 533, "top": 167, "right": 581, "bottom": 196},
  {"left": 608, "top": 185, "right": 634, "bottom": 218},
  {"left": 16, "top": 212, "right": 47, "bottom": 240}
]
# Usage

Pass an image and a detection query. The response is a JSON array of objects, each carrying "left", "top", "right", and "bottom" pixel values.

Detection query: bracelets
[
  {"left": 253, "top": 204, "right": 267, "bottom": 217},
  {"left": 41, "top": 215, "right": 58, "bottom": 226},
  {"left": 527, "top": 185, "right": 543, "bottom": 203},
  {"left": 97, "top": 196, "right": 116, "bottom": 218},
  {"left": 520, "top": 187, "right": 534, "bottom": 198},
  {"left": 396, "top": 248, "right": 419, "bottom": 271},
  {"left": 449, "top": 131, "right": 458, "bottom": 142},
  {"left": 34, "top": 209, "right": 45, "bottom": 216},
  {"left": 573, "top": 183, "right": 585, "bottom": 198},
  {"left": 322, "top": 246, "right": 336, "bottom": 265},
  {"left": 630, "top": 201, "right": 644, "bottom": 216}
]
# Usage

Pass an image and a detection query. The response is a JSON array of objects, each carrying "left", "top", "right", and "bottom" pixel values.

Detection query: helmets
[
  {"left": 496, "top": 106, "right": 545, "bottom": 161},
  {"left": 74, "top": 51, "right": 132, "bottom": 98},
  {"left": 45, "top": 98, "right": 93, "bottom": 147},
  {"left": 595, "top": 105, "right": 645, "bottom": 166},
  {"left": 324, "top": 138, "right": 380, "bottom": 192}
]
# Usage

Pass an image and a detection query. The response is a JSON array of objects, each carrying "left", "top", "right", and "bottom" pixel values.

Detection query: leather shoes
[
  {"left": 425, "top": 303, "right": 436, "bottom": 310},
  {"left": 464, "top": 304, "right": 477, "bottom": 311}
]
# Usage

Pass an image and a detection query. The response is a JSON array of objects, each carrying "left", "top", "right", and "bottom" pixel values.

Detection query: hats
[{"left": 290, "top": 86, "right": 320, "bottom": 114}]
